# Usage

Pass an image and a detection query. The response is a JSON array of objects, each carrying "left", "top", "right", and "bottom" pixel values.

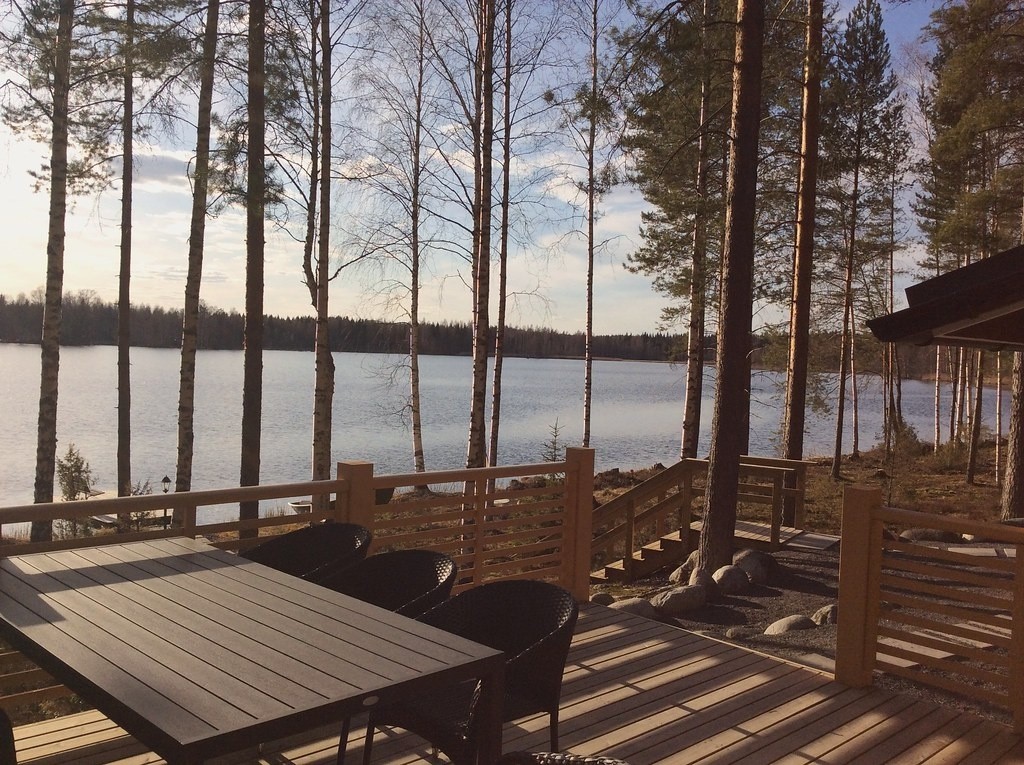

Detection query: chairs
[
  {"left": 307, "top": 549, "right": 457, "bottom": 763},
  {"left": 362, "top": 580, "right": 577, "bottom": 765},
  {"left": 239, "top": 521, "right": 372, "bottom": 580}
]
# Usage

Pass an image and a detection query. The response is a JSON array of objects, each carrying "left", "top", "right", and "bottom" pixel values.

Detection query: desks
[{"left": 0, "top": 536, "right": 503, "bottom": 765}]
